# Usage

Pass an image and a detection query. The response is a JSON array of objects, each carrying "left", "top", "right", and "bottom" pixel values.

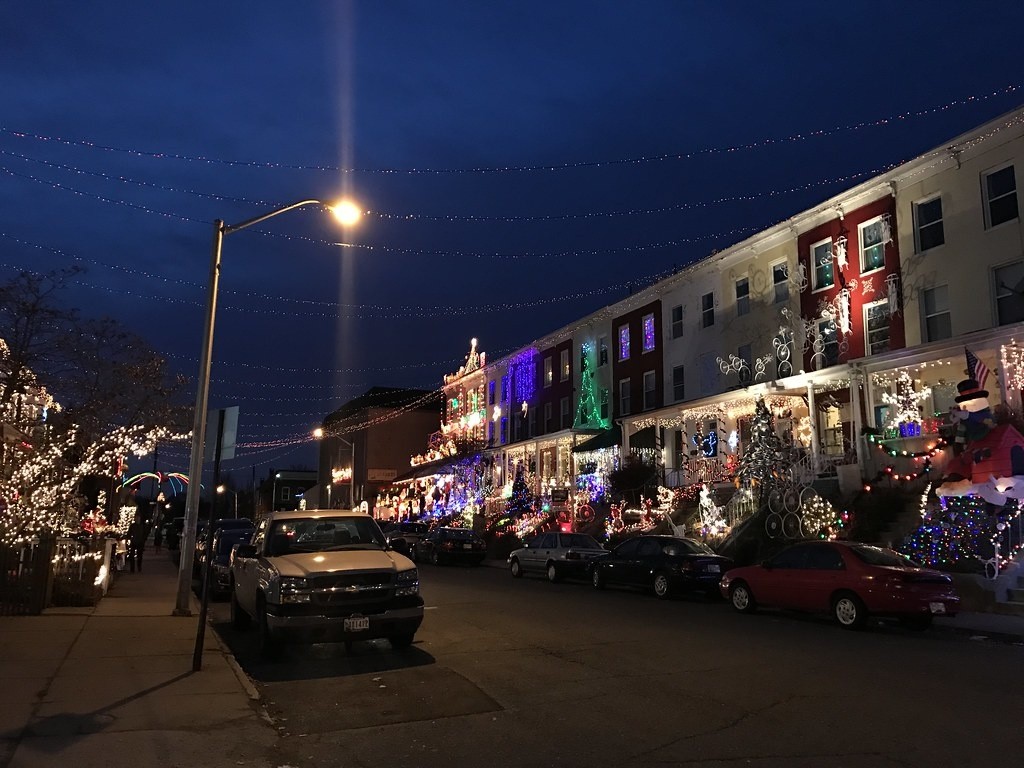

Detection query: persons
[
  {"left": 126, "top": 513, "right": 147, "bottom": 576},
  {"left": 154, "top": 524, "right": 164, "bottom": 555}
]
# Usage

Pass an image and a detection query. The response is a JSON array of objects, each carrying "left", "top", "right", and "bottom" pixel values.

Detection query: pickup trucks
[{"left": 229, "top": 508, "right": 424, "bottom": 643}]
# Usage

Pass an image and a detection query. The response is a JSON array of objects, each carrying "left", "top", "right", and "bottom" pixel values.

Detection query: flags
[{"left": 963, "top": 344, "right": 990, "bottom": 393}]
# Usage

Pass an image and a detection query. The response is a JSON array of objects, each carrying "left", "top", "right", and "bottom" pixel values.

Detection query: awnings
[{"left": 392, "top": 452, "right": 477, "bottom": 484}]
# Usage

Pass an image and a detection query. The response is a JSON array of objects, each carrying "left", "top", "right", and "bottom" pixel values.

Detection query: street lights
[
  {"left": 173, "top": 198, "right": 360, "bottom": 618},
  {"left": 219, "top": 485, "right": 237, "bottom": 518},
  {"left": 314, "top": 428, "right": 354, "bottom": 511}
]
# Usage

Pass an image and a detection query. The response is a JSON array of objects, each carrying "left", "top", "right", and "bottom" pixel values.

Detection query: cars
[
  {"left": 166, "top": 518, "right": 184, "bottom": 546},
  {"left": 411, "top": 527, "right": 488, "bottom": 564},
  {"left": 507, "top": 531, "right": 611, "bottom": 583},
  {"left": 193, "top": 518, "right": 258, "bottom": 598},
  {"left": 375, "top": 520, "right": 425, "bottom": 553},
  {"left": 719, "top": 539, "right": 960, "bottom": 628},
  {"left": 585, "top": 535, "right": 733, "bottom": 599}
]
